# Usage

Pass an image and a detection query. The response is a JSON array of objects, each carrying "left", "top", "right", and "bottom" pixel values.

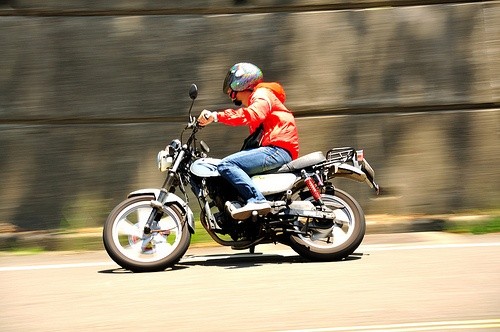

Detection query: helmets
[{"left": 223, "top": 62, "right": 263, "bottom": 96}]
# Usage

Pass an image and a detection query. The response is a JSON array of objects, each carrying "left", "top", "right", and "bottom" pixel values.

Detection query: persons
[{"left": 198, "top": 62, "right": 298, "bottom": 250}]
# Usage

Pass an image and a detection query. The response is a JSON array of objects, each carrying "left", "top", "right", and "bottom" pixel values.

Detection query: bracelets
[{"left": 213, "top": 112, "right": 217, "bottom": 122}]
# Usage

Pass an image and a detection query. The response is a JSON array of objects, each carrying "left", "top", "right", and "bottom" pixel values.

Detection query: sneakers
[
  {"left": 231, "top": 231, "right": 266, "bottom": 250},
  {"left": 231, "top": 199, "right": 272, "bottom": 220}
]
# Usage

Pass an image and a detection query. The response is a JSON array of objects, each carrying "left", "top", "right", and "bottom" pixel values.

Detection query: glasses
[{"left": 230, "top": 90, "right": 237, "bottom": 99}]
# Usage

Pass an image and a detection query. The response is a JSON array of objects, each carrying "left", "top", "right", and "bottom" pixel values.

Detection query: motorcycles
[{"left": 103, "top": 83, "right": 378, "bottom": 273}]
